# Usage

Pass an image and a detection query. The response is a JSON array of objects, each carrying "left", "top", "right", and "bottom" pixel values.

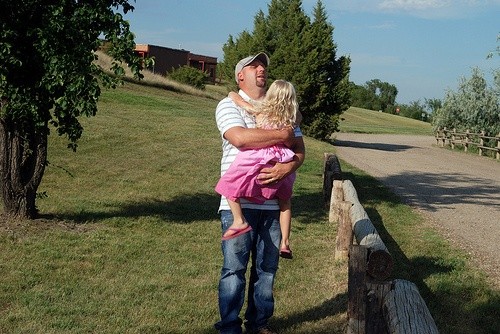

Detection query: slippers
[
  {"left": 221, "top": 226, "right": 252, "bottom": 241},
  {"left": 279, "top": 249, "right": 293, "bottom": 260}
]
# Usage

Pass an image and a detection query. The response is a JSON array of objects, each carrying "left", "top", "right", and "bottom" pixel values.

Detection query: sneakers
[{"left": 246, "top": 322, "right": 276, "bottom": 334}]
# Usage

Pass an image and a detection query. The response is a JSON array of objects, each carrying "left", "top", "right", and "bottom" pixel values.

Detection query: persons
[
  {"left": 214, "top": 78, "right": 299, "bottom": 334},
  {"left": 211, "top": 50, "right": 306, "bottom": 186}
]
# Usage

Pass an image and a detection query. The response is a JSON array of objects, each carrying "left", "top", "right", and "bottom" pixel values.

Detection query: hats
[{"left": 234, "top": 52, "right": 271, "bottom": 82}]
